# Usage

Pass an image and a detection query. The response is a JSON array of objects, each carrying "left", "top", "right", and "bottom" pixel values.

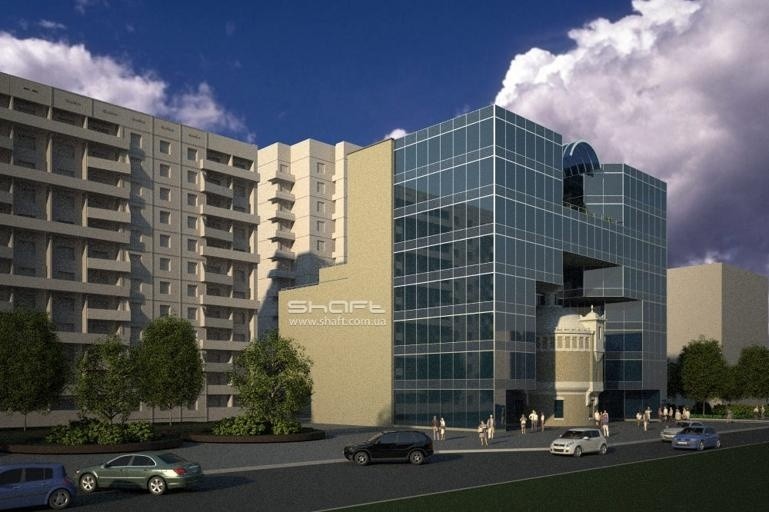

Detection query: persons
[
  {"left": 439, "top": 417, "right": 446, "bottom": 441},
  {"left": 540, "top": 412, "right": 546, "bottom": 432},
  {"left": 431, "top": 416, "right": 440, "bottom": 442},
  {"left": 487, "top": 414, "right": 495, "bottom": 440},
  {"left": 529, "top": 409, "right": 538, "bottom": 432},
  {"left": 635, "top": 406, "right": 653, "bottom": 432},
  {"left": 753, "top": 404, "right": 761, "bottom": 418},
  {"left": 761, "top": 405, "right": 766, "bottom": 419},
  {"left": 533, "top": 411, "right": 538, "bottom": 432},
  {"left": 519, "top": 413, "right": 528, "bottom": 436},
  {"left": 726, "top": 407, "right": 733, "bottom": 424},
  {"left": 478, "top": 420, "right": 488, "bottom": 448},
  {"left": 594, "top": 409, "right": 609, "bottom": 438},
  {"left": 657, "top": 406, "right": 690, "bottom": 423}
]
